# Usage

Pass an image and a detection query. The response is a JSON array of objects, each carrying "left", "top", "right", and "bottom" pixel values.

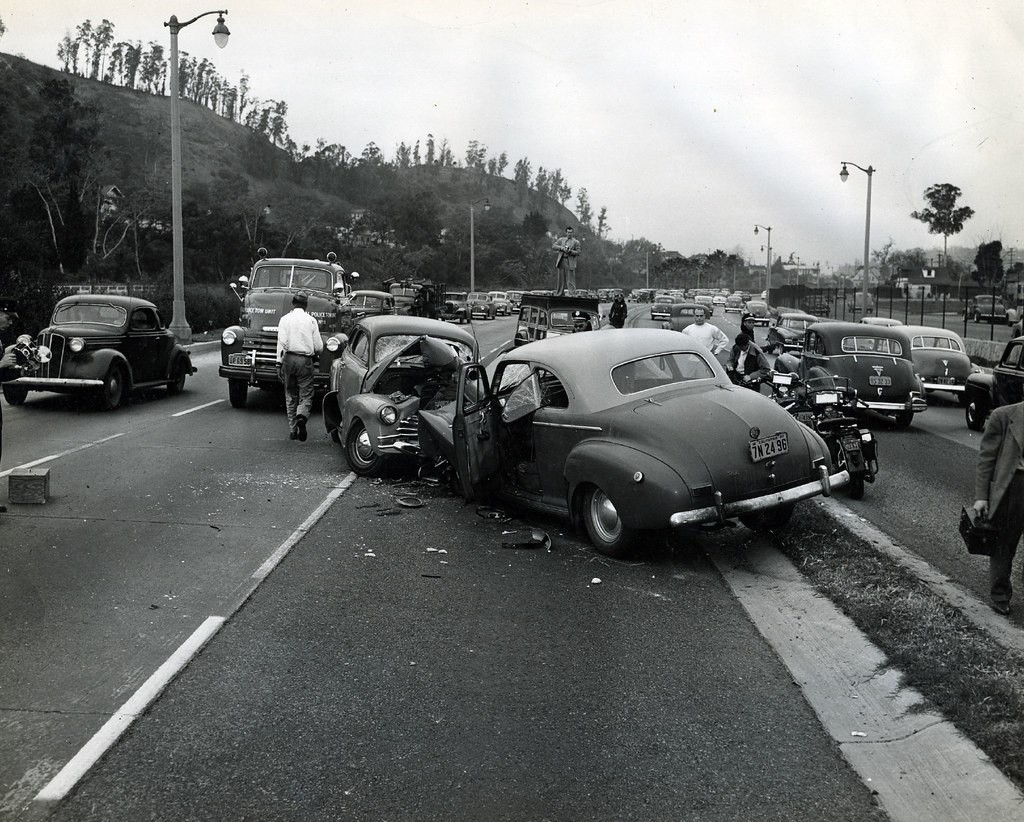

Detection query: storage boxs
[{"left": 7, "top": 468, "right": 50, "bottom": 504}]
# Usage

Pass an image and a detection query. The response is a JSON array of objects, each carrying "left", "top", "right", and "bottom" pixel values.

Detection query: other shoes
[
  {"left": 290, "top": 432, "right": 297, "bottom": 440},
  {"left": 297, "top": 418, "right": 307, "bottom": 442},
  {"left": 0, "top": 505, "right": 8, "bottom": 513}
]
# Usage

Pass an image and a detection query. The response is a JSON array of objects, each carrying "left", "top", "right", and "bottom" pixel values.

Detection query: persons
[
  {"left": 552, "top": 227, "right": 580, "bottom": 297},
  {"left": 726, "top": 312, "right": 771, "bottom": 393},
  {"left": 609, "top": 294, "right": 627, "bottom": 328},
  {"left": 973, "top": 401, "right": 1024, "bottom": 616},
  {"left": 571, "top": 310, "right": 592, "bottom": 333},
  {"left": 681, "top": 307, "right": 729, "bottom": 355},
  {"left": 276, "top": 290, "right": 323, "bottom": 442}
]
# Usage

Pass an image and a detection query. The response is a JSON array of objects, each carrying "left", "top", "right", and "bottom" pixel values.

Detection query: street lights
[
  {"left": 755, "top": 225, "right": 772, "bottom": 305},
  {"left": 471, "top": 197, "right": 490, "bottom": 292},
  {"left": 840, "top": 162, "right": 876, "bottom": 318},
  {"left": 164, "top": 10, "right": 230, "bottom": 340},
  {"left": 646, "top": 251, "right": 655, "bottom": 288}
]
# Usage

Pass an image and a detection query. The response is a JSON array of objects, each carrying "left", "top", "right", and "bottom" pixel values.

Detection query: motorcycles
[{"left": 768, "top": 377, "right": 880, "bottom": 498}]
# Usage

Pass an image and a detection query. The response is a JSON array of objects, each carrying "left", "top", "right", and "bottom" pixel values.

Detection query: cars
[
  {"left": 761, "top": 291, "right": 767, "bottom": 299},
  {"left": 684, "top": 288, "right": 731, "bottom": 299},
  {"left": 1, "top": 294, "right": 198, "bottom": 412},
  {"left": 442, "top": 291, "right": 533, "bottom": 323},
  {"left": 962, "top": 295, "right": 1024, "bottom": 339},
  {"left": 766, "top": 313, "right": 1024, "bottom": 431},
  {"left": 530, "top": 289, "right": 624, "bottom": 303},
  {"left": 650, "top": 295, "right": 711, "bottom": 331},
  {"left": 695, "top": 291, "right": 771, "bottom": 327},
  {"left": 453, "top": 292, "right": 851, "bottom": 558},
  {"left": 628, "top": 289, "right": 685, "bottom": 303},
  {"left": 847, "top": 292, "right": 874, "bottom": 312},
  {"left": 801, "top": 290, "right": 843, "bottom": 315}
]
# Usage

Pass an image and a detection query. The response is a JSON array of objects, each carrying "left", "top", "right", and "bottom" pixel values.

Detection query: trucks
[{"left": 219, "top": 247, "right": 493, "bottom": 477}]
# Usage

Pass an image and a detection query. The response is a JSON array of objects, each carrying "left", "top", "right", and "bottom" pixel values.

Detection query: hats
[
  {"left": 742, "top": 313, "right": 757, "bottom": 322},
  {"left": 617, "top": 294, "right": 623, "bottom": 298}
]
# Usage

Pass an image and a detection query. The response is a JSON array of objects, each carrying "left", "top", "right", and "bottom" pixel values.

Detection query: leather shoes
[{"left": 991, "top": 600, "right": 1011, "bottom": 616}]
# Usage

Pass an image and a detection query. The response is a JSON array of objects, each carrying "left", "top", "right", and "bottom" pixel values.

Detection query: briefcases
[{"left": 959, "top": 505, "right": 1000, "bottom": 558}]
